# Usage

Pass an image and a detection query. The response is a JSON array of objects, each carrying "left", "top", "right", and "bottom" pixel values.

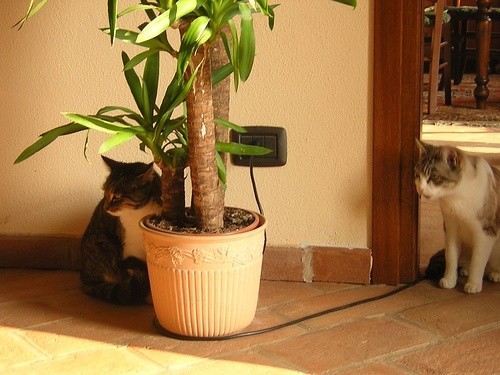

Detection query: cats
[
  {"left": 79, "top": 155, "right": 165, "bottom": 307},
  {"left": 412, "top": 138, "right": 499, "bottom": 294}
]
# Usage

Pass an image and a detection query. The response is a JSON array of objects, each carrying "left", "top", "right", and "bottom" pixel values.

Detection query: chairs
[{"left": 422, "top": 0, "right": 500, "bottom": 115}]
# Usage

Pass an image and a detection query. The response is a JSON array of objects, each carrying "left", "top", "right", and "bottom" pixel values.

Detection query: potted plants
[{"left": 11, "top": 0, "right": 359, "bottom": 338}]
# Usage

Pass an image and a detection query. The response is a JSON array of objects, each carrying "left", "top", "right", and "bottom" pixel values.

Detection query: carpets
[{"left": 423, "top": 76, "right": 500, "bottom": 128}]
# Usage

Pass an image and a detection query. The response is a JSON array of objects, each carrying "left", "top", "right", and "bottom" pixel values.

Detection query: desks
[{"left": 424, "top": 0, "right": 500, "bottom": 115}]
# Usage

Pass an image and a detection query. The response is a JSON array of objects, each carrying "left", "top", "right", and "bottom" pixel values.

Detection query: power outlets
[{"left": 231, "top": 126, "right": 288, "bottom": 167}]
